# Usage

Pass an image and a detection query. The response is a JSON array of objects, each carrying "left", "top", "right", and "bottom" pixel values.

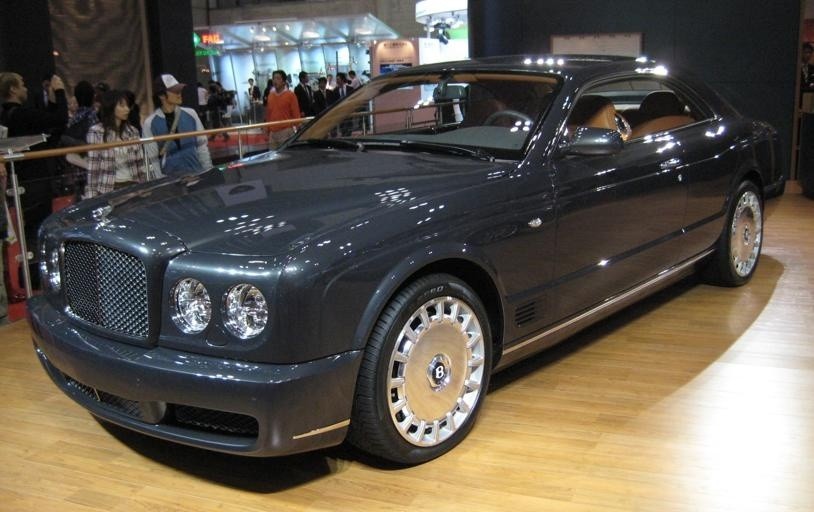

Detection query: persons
[
  {"left": 142, "top": 74, "right": 213, "bottom": 179},
  {"left": 265, "top": 70, "right": 301, "bottom": 151},
  {"left": 59, "top": 80, "right": 153, "bottom": 201},
  {"left": 248, "top": 78, "right": 261, "bottom": 101},
  {"left": 263, "top": 70, "right": 370, "bottom": 118},
  {"left": 197, "top": 79, "right": 230, "bottom": 142},
  {"left": 2, "top": 71, "right": 70, "bottom": 290}
]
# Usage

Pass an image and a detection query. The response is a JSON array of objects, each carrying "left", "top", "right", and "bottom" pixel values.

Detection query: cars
[{"left": 26, "top": 53, "right": 791, "bottom": 470}]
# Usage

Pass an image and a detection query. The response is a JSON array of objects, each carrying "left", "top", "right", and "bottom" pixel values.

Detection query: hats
[{"left": 153, "top": 73, "right": 188, "bottom": 94}]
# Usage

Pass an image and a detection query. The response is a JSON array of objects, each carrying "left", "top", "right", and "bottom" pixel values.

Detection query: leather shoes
[{"left": 7, "top": 291, "right": 26, "bottom": 303}]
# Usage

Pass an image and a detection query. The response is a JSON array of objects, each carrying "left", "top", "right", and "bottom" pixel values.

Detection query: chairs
[
  {"left": 463, "top": 99, "right": 512, "bottom": 127},
  {"left": 572, "top": 96, "right": 617, "bottom": 131},
  {"left": 638, "top": 92, "right": 682, "bottom": 120}
]
